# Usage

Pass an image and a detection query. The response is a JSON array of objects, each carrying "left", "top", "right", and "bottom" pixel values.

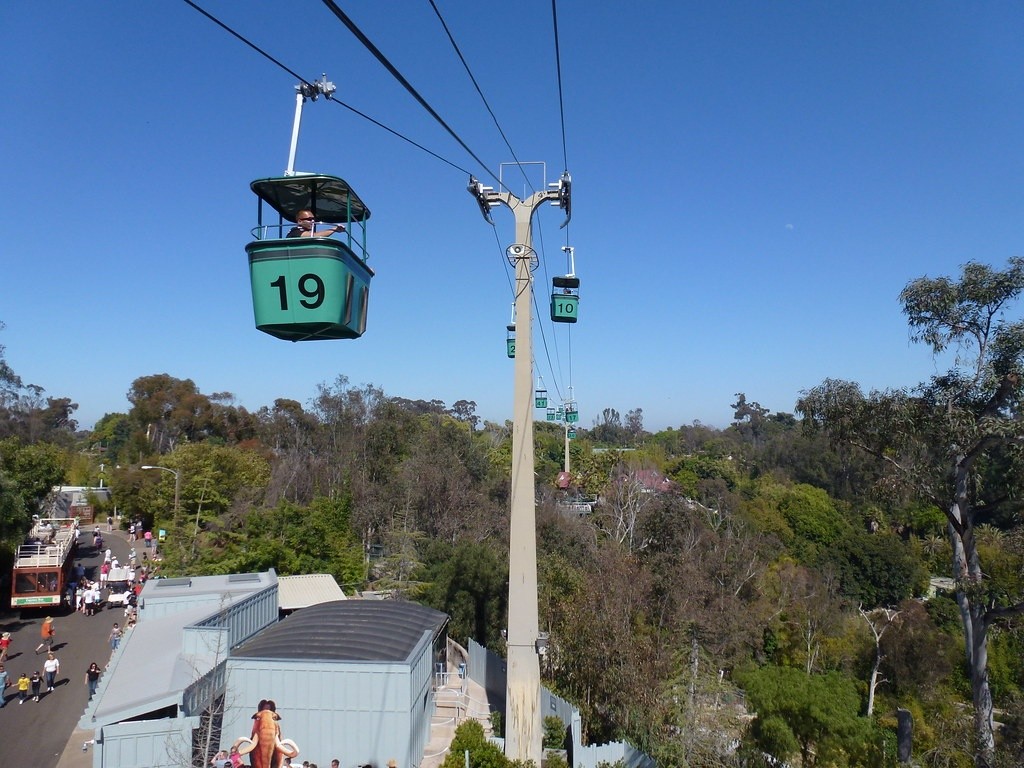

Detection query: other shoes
[
  {"left": 48, "top": 651, "right": 55, "bottom": 654},
  {"left": 46, "top": 687, "right": 51, "bottom": 692},
  {"left": 51, "top": 686, "right": 55, "bottom": 692},
  {"left": 19, "top": 699, "right": 23, "bottom": 705},
  {"left": 88, "top": 697, "right": 93, "bottom": 702},
  {"left": 35, "top": 649, "right": 39, "bottom": 657},
  {"left": 32, "top": 696, "right": 36, "bottom": 701},
  {"left": 35, "top": 695, "right": 40, "bottom": 702}
]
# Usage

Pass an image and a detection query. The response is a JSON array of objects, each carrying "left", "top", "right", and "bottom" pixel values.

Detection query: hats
[
  {"left": 2, "top": 632, "right": 11, "bottom": 641},
  {"left": 44, "top": 616, "right": 54, "bottom": 624}
]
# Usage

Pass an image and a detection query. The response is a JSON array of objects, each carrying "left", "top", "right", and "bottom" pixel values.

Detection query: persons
[
  {"left": 25, "top": 527, "right": 56, "bottom": 550},
  {"left": 0, "top": 632, "right": 15, "bottom": 664},
  {"left": 0, "top": 665, "right": 10, "bottom": 709},
  {"left": 362, "top": 764, "right": 372, "bottom": 768},
  {"left": 211, "top": 746, "right": 252, "bottom": 768},
  {"left": 85, "top": 662, "right": 102, "bottom": 700},
  {"left": 286, "top": 209, "right": 346, "bottom": 238},
  {"left": 107, "top": 623, "right": 123, "bottom": 652},
  {"left": 35, "top": 616, "right": 56, "bottom": 655},
  {"left": 42, "top": 652, "right": 60, "bottom": 691},
  {"left": 331, "top": 759, "right": 340, "bottom": 768},
  {"left": 64, "top": 515, "right": 167, "bottom": 630},
  {"left": 50, "top": 577, "right": 58, "bottom": 591},
  {"left": 283, "top": 758, "right": 293, "bottom": 768},
  {"left": 563, "top": 288, "right": 571, "bottom": 294},
  {"left": 387, "top": 760, "right": 398, "bottom": 768},
  {"left": 39, "top": 520, "right": 72, "bottom": 530},
  {"left": 11, "top": 671, "right": 44, "bottom": 705},
  {"left": 303, "top": 761, "right": 317, "bottom": 768}
]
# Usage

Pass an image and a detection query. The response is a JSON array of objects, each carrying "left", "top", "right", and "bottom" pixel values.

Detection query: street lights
[{"left": 141, "top": 465, "right": 178, "bottom": 529}]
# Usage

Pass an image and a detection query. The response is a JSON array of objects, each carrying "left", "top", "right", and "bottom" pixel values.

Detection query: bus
[{"left": 10, "top": 515, "right": 81, "bottom": 617}]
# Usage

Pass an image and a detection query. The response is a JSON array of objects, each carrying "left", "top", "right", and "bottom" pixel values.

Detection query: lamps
[{"left": 82, "top": 740, "right": 94, "bottom": 753}]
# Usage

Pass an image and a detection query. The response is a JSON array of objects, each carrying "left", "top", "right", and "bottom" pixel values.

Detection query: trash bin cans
[{"left": 459, "top": 662, "right": 467, "bottom": 678}]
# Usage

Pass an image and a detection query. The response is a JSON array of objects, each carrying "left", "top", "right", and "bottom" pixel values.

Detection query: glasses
[{"left": 300, "top": 217, "right": 315, "bottom": 221}]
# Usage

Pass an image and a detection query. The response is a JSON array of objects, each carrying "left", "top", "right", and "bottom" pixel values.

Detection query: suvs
[{"left": 106, "top": 569, "right": 133, "bottom": 610}]
